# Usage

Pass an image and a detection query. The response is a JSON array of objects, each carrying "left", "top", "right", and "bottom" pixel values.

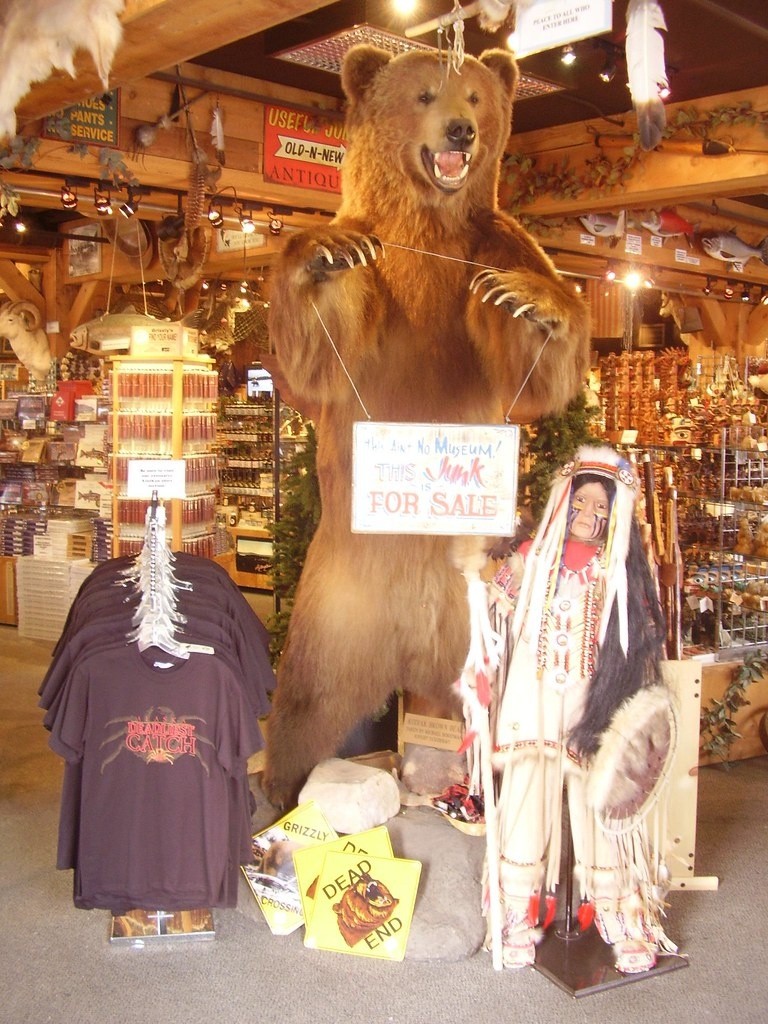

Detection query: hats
[{"left": 560, "top": 443, "right": 641, "bottom": 486}]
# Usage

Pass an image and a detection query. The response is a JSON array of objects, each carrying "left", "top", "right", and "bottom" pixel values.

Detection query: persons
[{"left": 468, "top": 450, "right": 682, "bottom": 970}]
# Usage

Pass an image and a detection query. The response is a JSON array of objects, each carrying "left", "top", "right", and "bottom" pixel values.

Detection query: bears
[{"left": 254, "top": 44, "right": 592, "bottom": 814}]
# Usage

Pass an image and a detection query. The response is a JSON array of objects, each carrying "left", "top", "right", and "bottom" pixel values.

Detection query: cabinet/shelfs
[{"left": 1, "top": 345, "right": 768, "bottom": 681}]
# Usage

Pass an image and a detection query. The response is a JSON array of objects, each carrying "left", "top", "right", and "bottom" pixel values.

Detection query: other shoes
[
  {"left": 497, "top": 920, "right": 535, "bottom": 970},
  {"left": 611, "top": 936, "right": 658, "bottom": 974}
]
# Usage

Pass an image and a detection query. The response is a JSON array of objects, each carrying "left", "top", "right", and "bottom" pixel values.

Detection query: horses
[
  {"left": 659, "top": 291, "right": 685, "bottom": 331},
  {"left": 200, "top": 293, "right": 250, "bottom": 348}
]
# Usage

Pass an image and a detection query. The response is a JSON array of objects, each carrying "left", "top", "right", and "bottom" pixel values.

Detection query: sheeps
[{"left": 0, "top": 300, "right": 52, "bottom": 382}]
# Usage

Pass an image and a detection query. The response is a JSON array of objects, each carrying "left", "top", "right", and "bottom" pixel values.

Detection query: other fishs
[
  {"left": 701, "top": 226, "right": 768, "bottom": 274},
  {"left": 640, "top": 204, "right": 701, "bottom": 249},
  {"left": 69, "top": 309, "right": 204, "bottom": 352},
  {"left": 78, "top": 490, "right": 100, "bottom": 502},
  {"left": 579, "top": 209, "right": 635, "bottom": 238},
  {"left": 80, "top": 448, "right": 105, "bottom": 460}
]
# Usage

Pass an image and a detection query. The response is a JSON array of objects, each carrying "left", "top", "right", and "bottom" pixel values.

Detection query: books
[{"left": 3, "top": 393, "right": 115, "bottom": 645}]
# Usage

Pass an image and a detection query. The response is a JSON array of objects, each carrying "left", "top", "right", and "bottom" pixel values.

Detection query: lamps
[
  {"left": 239, "top": 216, "right": 254, "bottom": 234},
  {"left": 119, "top": 199, "right": 139, "bottom": 219},
  {"left": 207, "top": 201, "right": 224, "bottom": 228},
  {"left": 599, "top": 63, "right": 617, "bottom": 82},
  {"left": 724, "top": 288, "right": 734, "bottom": 299},
  {"left": 741, "top": 292, "right": 750, "bottom": 302},
  {"left": 760, "top": 296, "right": 768, "bottom": 305},
  {"left": 645, "top": 276, "right": 656, "bottom": 288},
  {"left": 604, "top": 268, "right": 616, "bottom": 281},
  {"left": 702, "top": 285, "right": 712, "bottom": 296},
  {"left": 60, "top": 186, "right": 78, "bottom": 210},
  {"left": 269, "top": 218, "right": 283, "bottom": 236},
  {"left": 15, "top": 223, "right": 26, "bottom": 233},
  {"left": 94, "top": 194, "right": 112, "bottom": 216},
  {"left": 561, "top": 44, "right": 577, "bottom": 65}
]
